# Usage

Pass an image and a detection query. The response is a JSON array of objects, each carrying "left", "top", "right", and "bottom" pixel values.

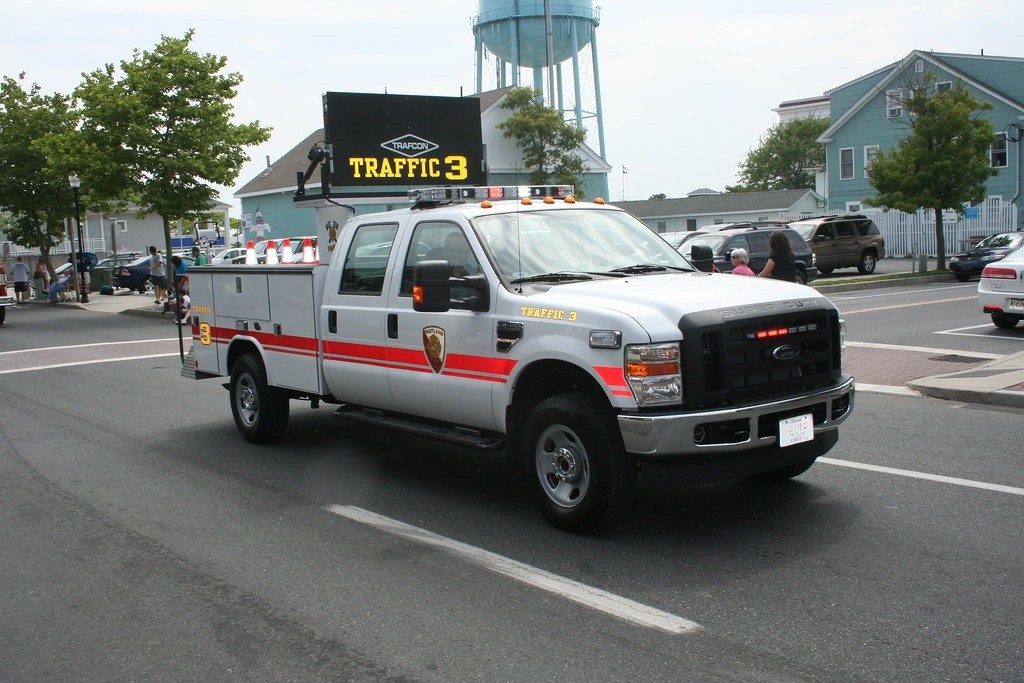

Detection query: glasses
[{"left": 731, "top": 256, "right": 735, "bottom": 259}]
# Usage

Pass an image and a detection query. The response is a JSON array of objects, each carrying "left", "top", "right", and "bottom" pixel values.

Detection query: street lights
[
  {"left": 69, "top": 176, "right": 90, "bottom": 303},
  {"left": 80, "top": 222, "right": 85, "bottom": 252}
]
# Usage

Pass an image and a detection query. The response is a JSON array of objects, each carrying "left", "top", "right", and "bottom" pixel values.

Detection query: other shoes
[
  {"left": 154, "top": 300, "right": 161, "bottom": 304},
  {"left": 22, "top": 301, "right": 29, "bottom": 304},
  {"left": 174, "top": 319, "right": 187, "bottom": 325},
  {"left": 17, "top": 301, "right": 22, "bottom": 304}
]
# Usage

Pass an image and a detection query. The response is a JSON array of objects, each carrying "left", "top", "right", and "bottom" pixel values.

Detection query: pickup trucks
[{"left": 174, "top": 92, "right": 857, "bottom": 533}]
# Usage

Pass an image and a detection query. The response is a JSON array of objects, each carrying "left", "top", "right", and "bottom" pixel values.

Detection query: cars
[
  {"left": 0, "top": 258, "right": 14, "bottom": 325},
  {"left": 211, "top": 248, "right": 247, "bottom": 265},
  {"left": 111, "top": 254, "right": 197, "bottom": 294},
  {"left": 977, "top": 243, "right": 1024, "bottom": 328},
  {"left": 232, "top": 239, "right": 288, "bottom": 265},
  {"left": 258, "top": 236, "right": 318, "bottom": 265},
  {"left": 97, "top": 253, "right": 140, "bottom": 267},
  {"left": 356, "top": 241, "right": 433, "bottom": 255},
  {"left": 948, "top": 231, "right": 1024, "bottom": 282}
]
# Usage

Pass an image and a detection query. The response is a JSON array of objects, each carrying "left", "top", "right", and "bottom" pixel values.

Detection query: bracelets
[{"left": 462, "top": 298, "right": 469, "bottom": 308}]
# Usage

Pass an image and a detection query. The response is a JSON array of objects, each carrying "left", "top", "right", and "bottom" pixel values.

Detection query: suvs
[
  {"left": 675, "top": 221, "right": 817, "bottom": 286},
  {"left": 786, "top": 215, "right": 886, "bottom": 275}
]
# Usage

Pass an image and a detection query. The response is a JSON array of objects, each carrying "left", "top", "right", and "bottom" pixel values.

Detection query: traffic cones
[{"left": 246, "top": 237, "right": 320, "bottom": 266}]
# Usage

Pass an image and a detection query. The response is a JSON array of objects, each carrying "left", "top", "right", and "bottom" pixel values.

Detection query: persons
[
  {"left": 37, "top": 256, "right": 74, "bottom": 304},
  {"left": 444, "top": 233, "right": 484, "bottom": 309},
  {"left": 9, "top": 257, "right": 31, "bottom": 304},
  {"left": 157, "top": 247, "right": 206, "bottom": 326},
  {"left": 148, "top": 247, "right": 166, "bottom": 305},
  {"left": 757, "top": 231, "right": 796, "bottom": 282},
  {"left": 730, "top": 248, "right": 756, "bottom": 276}
]
